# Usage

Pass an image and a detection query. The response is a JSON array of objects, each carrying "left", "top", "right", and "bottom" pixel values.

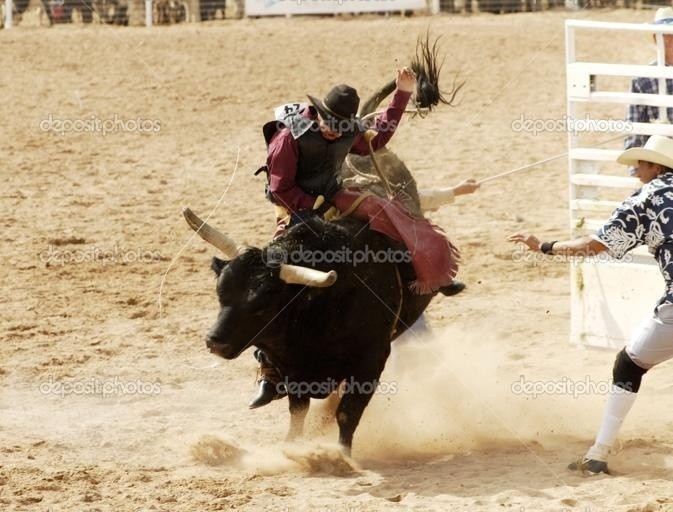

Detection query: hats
[
  {"left": 617, "top": 133, "right": 673, "bottom": 170},
  {"left": 306, "top": 84, "right": 361, "bottom": 137},
  {"left": 642, "top": 6, "right": 672, "bottom": 44}
]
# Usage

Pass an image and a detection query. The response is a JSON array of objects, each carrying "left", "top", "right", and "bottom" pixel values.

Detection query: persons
[
  {"left": 262, "top": 68, "right": 466, "bottom": 297},
  {"left": 622, "top": 6, "right": 673, "bottom": 177},
  {"left": 418, "top": 177, "right": 480, "bottom": 213},
  {"left": 505, "top": 132, "right": 671, "bottom": 476},
  {"left": 43, "top": 0, "right": 227, "bottom": 25}
]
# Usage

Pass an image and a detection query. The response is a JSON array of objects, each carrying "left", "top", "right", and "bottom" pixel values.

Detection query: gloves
[{"left": 313, "top": 196, "right": 339, "bottom": 222}]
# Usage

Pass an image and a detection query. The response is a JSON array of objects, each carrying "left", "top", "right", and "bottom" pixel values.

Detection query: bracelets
[{"left": 541, "top": 241, "right": 559, "bottom": 256}]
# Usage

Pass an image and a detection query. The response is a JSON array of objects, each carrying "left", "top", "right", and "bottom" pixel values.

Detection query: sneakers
[{"left": 567, "top": 456, "right": 608, "bottom": 475}]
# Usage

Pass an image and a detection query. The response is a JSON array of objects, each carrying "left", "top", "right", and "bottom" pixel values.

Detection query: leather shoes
[
  {"left": 438, "top": 278, "right": 465, "bottom": 297},
  {"left": 248, "top": 379, "right": 287, "bottom": 409}
]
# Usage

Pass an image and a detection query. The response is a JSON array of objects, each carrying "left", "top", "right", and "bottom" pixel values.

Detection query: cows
[{"left": 182, "top": 23, "right": 468, "bottom": 462}]
